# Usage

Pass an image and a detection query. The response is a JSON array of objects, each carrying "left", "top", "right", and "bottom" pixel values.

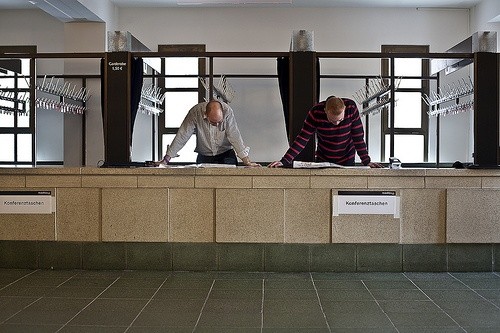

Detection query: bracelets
[{"left": 163, "top": 157, "right": 169, "bottom": 163}]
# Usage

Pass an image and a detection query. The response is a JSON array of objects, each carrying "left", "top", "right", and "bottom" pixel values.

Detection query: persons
[
  {"left": 267, "top": 96, "right": 386, "bottom": 168},
  {"left": 152, "top": 101, "right": 262, "bottom": 167}
]
